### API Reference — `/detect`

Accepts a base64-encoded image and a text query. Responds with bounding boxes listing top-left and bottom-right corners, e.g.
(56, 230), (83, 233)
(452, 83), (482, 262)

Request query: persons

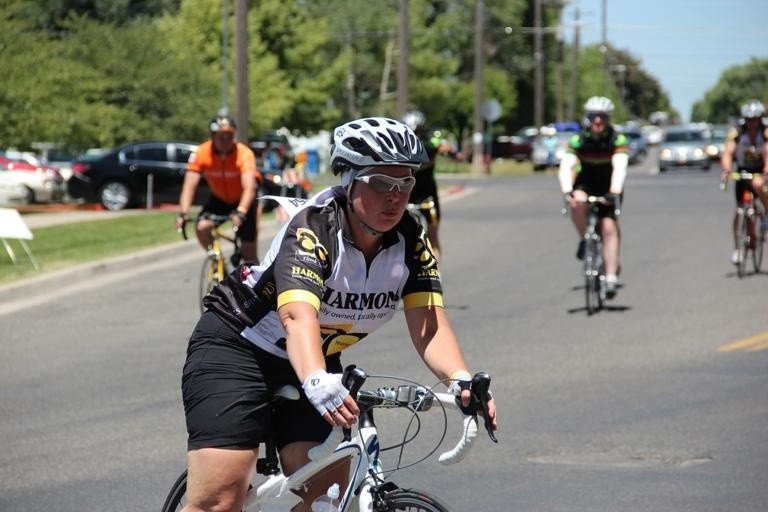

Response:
(399, 110), (444, 283)
(178, 117), (497, 511)
(174, 112), (260, 267)
(556, 94), (630, 300)
(718, 98), (768, 265)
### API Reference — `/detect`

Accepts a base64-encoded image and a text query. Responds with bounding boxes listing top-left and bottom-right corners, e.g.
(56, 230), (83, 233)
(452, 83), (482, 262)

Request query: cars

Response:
(465, 118), (729, 175)
(67, 140), (306, 212)
(0, 147), (75, 210)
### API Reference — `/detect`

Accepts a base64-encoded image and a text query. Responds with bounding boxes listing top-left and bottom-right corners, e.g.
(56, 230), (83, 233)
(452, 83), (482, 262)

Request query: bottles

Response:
(309, 482), (343, 512)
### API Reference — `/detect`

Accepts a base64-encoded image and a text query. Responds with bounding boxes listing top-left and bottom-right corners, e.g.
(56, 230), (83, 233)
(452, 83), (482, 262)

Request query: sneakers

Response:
(577, 241), (586, 259)
(732, 250), (744, 264)
(605, 282), (617, 300)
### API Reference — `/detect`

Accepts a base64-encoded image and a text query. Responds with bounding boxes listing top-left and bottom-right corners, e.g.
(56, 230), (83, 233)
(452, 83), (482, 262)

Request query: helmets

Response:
(585, 96), (615, 114)
(329, 111), (430, 177)
(739, 100), (764, 119)
(210, 117), (237, 132)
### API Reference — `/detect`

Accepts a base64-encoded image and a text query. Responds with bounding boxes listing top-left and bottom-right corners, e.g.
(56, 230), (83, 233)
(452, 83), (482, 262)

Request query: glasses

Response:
(353, 175), (416, 195)
(587, 113), (608, 121)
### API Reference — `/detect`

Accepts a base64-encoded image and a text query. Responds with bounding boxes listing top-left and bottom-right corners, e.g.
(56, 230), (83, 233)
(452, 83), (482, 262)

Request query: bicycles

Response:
(179, 213), (242, 317)
(718, 169), (768, 279)
(162, 365), (497, 512)
(560, 194), (622, 316)
